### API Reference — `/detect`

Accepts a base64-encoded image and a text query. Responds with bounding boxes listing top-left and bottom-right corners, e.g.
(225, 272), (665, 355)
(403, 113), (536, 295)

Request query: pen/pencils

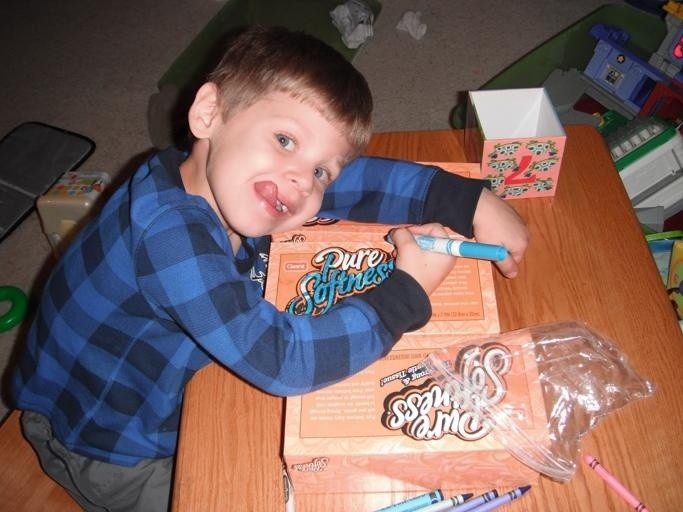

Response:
(384, 234), (508, 261)
(583, 454), (649, 512)
(374, 485), (531, 512)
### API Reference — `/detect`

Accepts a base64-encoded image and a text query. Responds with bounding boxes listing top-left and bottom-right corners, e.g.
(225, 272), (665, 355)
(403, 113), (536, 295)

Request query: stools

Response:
(0, 407), (87, 512)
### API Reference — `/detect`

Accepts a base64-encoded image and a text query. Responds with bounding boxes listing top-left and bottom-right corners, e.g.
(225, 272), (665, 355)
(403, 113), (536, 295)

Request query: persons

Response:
(1, 21), (531, 511)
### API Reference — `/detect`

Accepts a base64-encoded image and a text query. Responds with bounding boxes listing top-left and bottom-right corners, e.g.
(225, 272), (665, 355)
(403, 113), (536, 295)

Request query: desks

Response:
(170, 126), (683, 512)
